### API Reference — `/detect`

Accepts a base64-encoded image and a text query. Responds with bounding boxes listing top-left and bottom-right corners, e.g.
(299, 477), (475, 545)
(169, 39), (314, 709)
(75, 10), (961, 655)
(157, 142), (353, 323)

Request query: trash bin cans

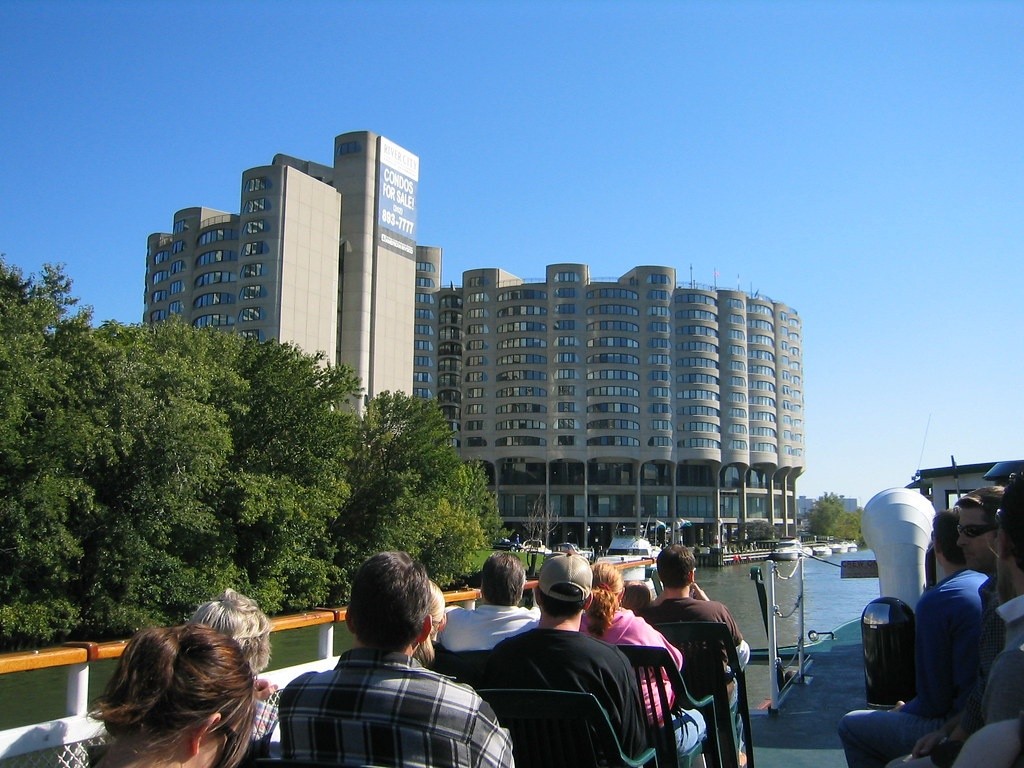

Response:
(860, 597), (916, 711)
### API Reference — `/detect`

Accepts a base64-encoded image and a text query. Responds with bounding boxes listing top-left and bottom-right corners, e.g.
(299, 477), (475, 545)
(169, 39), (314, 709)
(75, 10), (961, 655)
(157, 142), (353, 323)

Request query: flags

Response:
(656, 521), (667, 529)
(680, 519), (691, 527)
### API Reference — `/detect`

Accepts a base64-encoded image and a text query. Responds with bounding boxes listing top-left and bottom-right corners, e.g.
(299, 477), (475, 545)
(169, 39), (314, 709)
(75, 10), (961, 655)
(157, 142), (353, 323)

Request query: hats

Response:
(538, 549), (595, 603)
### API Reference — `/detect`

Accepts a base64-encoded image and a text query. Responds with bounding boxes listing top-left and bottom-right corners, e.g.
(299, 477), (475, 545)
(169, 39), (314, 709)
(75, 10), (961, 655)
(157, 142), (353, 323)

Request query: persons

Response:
(644, 544), (750, 768)
(622, 582), (651, 616)
(580, 562), (706, 755)
(278, 551), (515, 768)
(415, 579), (480, 689)
(91, 623), (255, 768)
(191, 589), (278, 756)
(836, 473), (1024, 768)
(480, 551), (648, 768)
(439, 551), (541, 651)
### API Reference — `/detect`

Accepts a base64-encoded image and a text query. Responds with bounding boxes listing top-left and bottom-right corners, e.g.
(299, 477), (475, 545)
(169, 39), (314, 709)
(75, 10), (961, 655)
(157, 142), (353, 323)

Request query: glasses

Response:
(930, 530), (943, 560)
(688, 567), (697, 575)
(956, 522), (999, 538)
(217, 722), (239, 767)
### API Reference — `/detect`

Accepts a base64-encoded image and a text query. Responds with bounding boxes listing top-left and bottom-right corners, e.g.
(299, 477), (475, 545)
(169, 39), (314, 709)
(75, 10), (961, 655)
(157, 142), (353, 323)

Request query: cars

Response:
(493, 537), (522, 553)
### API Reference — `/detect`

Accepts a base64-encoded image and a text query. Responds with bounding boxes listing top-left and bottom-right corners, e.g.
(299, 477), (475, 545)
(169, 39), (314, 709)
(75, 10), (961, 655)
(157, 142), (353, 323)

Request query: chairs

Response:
(475, 688), (659, 768)
(238, 758), (391, 768)
(613, 645), (723, 768)
(427, 650), (491, 690)
(652, 621), (754, 768)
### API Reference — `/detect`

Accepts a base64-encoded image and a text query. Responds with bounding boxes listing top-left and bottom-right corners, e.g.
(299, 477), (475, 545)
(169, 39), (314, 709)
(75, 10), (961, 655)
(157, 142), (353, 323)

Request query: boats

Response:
(829, 541), (858, 553)
(596, 536), (696, 589)
(523, 540), (552, 556)
(552, 542), (592, 559)
(771, 539), (812, 561)
(813, 543), (833, 556)
